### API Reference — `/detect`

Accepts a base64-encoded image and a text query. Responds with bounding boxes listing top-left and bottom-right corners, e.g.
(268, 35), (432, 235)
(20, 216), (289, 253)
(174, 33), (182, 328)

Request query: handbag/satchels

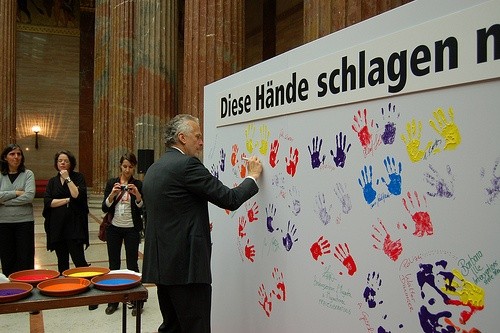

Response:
(98, 202), (117, 242)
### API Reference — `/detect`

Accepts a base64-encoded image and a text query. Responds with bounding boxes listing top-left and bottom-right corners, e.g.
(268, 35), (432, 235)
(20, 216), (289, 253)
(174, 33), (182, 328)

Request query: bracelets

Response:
(65, 198), (68, 204)
(65, 177), (71, 183)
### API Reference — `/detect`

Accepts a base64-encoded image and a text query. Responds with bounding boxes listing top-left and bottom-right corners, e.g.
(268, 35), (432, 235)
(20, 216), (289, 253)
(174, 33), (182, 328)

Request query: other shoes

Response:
(132, 305), (143, 316)
(89, 304), (99, 310)
(105, 305), (117, 315)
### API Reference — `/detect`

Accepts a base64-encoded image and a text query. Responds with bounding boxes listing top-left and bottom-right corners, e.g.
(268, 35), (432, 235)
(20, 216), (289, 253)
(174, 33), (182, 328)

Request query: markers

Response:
(241, 157), (248, 161)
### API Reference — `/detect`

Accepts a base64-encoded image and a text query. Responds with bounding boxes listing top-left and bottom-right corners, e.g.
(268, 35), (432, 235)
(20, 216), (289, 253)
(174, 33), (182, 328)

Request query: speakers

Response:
(137, 149), (154, 174)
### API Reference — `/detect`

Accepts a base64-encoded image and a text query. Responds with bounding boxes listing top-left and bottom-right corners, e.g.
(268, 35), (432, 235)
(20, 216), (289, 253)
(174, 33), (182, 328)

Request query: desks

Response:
(0, 276), (148, 333)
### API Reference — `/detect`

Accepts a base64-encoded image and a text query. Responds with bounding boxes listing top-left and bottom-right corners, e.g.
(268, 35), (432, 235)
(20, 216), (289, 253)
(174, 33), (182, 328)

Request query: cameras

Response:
(119, 185), (131, 192)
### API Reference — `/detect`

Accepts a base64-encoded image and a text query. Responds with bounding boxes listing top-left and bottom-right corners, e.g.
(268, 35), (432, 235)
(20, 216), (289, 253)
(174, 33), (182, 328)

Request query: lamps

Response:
(32, 126), (41, 149)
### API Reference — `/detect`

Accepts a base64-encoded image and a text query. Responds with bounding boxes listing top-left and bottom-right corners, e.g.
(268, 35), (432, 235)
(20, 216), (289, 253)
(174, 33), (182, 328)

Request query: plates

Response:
(9, 269), (60, 287)
(36, 278), (91, 297)
(62, 267), (110, 280)
(90, 274), (141, 290)
(0, 282), (33, 303)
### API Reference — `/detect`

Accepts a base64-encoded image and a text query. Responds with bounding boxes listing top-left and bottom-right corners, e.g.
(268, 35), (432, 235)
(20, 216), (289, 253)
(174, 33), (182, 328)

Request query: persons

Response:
(42, 150), (99, 310)
(102, 153), (143, 316)
(141, 113), (262, 333)
(0, 143), (41, 314)
